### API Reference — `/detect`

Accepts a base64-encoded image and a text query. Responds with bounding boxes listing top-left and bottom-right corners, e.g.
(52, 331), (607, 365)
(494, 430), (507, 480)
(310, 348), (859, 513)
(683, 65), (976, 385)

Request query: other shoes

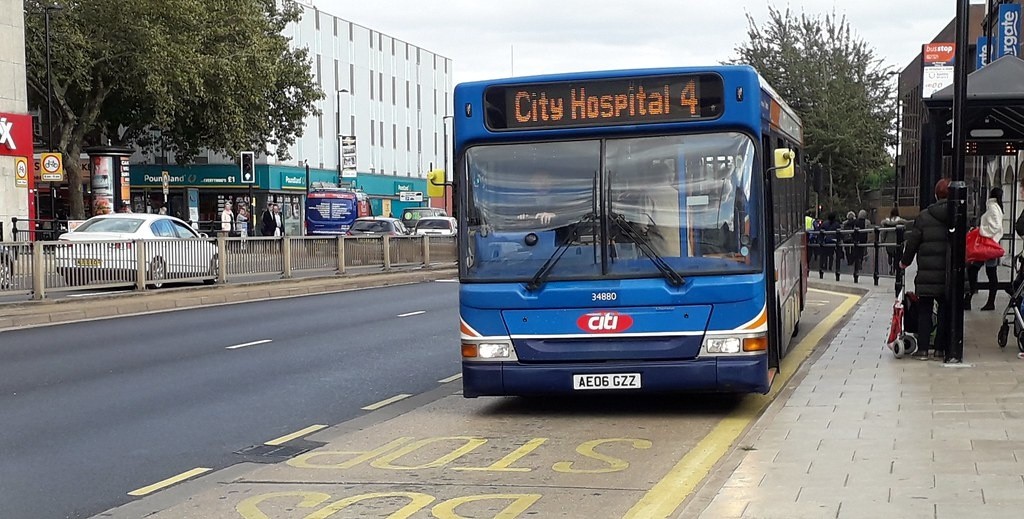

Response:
(934, 350), (944, 361)
(912, 350), (928, 361)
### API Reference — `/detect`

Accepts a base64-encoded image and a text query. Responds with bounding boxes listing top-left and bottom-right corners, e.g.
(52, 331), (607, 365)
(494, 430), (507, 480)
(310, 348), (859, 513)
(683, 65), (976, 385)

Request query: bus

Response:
(426, 62), (811, 400)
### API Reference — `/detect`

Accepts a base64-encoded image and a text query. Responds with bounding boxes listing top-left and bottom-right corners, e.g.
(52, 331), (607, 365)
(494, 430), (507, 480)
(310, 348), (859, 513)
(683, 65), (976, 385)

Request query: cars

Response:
(53, 211), (229, 290)
(0, 243), (15, 289)
(345, 215), (409, 236)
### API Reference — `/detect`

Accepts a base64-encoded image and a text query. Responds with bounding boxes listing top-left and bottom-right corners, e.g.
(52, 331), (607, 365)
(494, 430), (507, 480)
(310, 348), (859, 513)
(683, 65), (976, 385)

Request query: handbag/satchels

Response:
(965, 229), (1005, 262)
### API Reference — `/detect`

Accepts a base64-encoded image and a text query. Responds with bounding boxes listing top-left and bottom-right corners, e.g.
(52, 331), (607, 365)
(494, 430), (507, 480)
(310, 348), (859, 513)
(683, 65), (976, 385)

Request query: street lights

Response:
(337, 88), (350, 190)
(45, 4), (64, 250)
(442, 115), (453, 214)
(887, 70), (902, 222)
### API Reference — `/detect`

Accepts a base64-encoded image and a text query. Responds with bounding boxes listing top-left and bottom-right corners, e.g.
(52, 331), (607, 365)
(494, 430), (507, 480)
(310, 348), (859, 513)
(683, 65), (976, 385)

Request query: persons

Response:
(517, 169), (558, 224)
(805, 210), (819, 262)
(817, 211), (840, 271)
(237, 206), (250, 235)
(841, 209), (872, 265)
(882, 208), (902, 274)
(900, 178), (951, 360)
(220, 203), (235, 230)
(158, 207), (166, 215)
(964, 187), (1004, 311)
(263, 204), (285, 236)
(630, 162), (691, 256)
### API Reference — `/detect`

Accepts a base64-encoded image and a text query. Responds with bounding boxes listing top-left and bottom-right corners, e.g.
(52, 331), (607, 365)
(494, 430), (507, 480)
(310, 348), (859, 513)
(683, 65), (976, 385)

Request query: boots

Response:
(963, 262), (981, 310)
(980, 266), (998, 311)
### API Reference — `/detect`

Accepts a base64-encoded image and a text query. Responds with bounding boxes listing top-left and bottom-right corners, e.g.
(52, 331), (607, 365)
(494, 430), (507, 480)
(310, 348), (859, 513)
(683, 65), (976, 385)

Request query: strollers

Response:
(996, 248), (1024, 354)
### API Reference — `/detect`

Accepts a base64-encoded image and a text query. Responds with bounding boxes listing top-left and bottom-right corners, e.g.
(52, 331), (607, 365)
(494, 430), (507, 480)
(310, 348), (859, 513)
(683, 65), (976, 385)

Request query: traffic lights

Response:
(240, 151), (256, 183)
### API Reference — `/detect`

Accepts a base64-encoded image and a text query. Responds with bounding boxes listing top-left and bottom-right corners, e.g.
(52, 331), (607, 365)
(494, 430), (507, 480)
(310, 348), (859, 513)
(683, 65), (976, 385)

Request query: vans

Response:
(413, 216), (458, 236)
(400, 206), (447, 236)
